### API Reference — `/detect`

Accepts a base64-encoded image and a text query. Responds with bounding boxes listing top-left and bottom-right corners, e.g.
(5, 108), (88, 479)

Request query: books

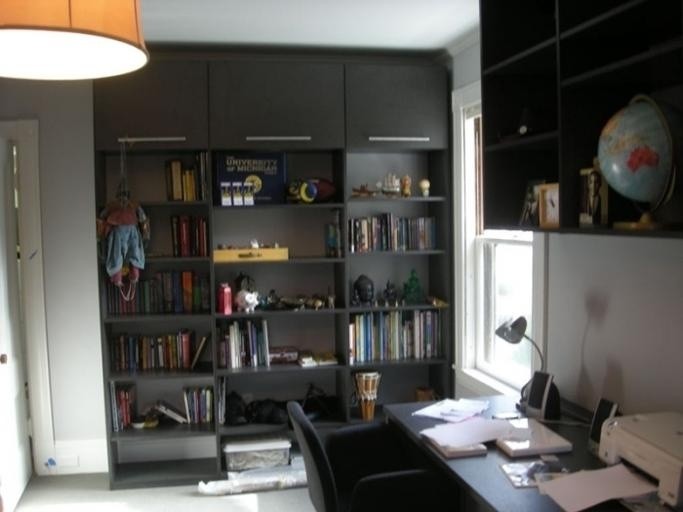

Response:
(216, 318), (271, 369)
(159, 155), (214, 429)
(424, 417), (487, 459)
(347, 308), (444, 368)
(348, 213), (438, 255)
(99, 269), (159, 434)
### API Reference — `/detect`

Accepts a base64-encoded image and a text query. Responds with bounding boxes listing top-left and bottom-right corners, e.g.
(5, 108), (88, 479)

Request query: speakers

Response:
(526, 372), (561, 421)
(587, 398), (622, 456)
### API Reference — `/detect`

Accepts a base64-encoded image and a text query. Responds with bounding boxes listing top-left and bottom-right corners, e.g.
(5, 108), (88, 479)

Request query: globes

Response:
(599, 94), (681, 231)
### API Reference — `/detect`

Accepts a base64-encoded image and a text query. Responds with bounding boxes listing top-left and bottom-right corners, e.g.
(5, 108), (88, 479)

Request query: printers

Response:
(599, 409), (682, 511)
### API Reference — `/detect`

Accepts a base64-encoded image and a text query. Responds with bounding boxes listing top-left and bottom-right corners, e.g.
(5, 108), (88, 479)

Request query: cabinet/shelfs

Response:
(478, 0), (683, 239)
(93, 51), (458, 490)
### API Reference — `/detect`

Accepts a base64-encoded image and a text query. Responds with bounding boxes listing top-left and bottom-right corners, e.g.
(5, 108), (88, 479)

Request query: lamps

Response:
(0, 1), (151, 84)
(494, 315), (548, 412)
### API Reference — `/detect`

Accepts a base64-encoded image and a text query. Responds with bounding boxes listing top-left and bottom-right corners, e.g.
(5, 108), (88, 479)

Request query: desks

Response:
(382, 397), (673, 512)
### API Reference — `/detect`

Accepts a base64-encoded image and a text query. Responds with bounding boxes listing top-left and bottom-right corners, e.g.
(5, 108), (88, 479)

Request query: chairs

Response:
(284, 400), (454, 512)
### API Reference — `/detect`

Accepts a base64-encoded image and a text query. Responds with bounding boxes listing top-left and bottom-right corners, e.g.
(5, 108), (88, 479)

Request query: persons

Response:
(582, 170), (602, 224)
(96, 180), (154, 302)
